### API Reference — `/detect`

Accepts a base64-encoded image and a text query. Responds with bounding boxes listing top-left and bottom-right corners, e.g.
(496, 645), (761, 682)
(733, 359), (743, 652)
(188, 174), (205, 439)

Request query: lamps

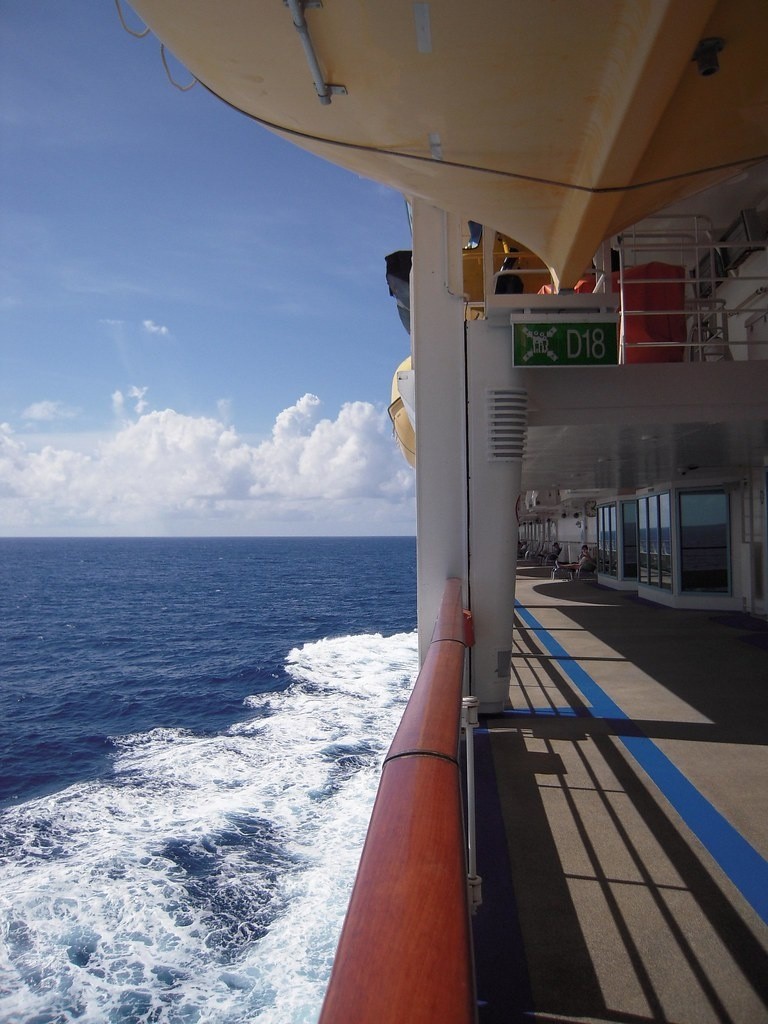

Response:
(692, 37), (723, 76)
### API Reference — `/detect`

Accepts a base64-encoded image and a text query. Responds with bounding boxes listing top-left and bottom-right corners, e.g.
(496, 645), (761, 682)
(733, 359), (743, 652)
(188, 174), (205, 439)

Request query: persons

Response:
(517, 540), (528, 556)
(537, 543), (561, 565)
(555, 545), (594, 570)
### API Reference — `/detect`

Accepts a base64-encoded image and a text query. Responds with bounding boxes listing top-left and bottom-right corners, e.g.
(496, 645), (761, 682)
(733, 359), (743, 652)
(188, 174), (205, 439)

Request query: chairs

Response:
(542, 553), (559, 566)
(551, 557), (597, 579)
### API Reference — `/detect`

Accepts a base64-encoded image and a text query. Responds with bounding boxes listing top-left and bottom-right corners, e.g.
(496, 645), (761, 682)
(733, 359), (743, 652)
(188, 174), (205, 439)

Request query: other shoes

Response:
(555, 560), (559, 569)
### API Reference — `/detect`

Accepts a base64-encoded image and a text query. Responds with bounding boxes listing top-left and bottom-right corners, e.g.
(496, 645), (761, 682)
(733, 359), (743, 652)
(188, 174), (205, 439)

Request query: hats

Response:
(552, 542), (558, 546)
